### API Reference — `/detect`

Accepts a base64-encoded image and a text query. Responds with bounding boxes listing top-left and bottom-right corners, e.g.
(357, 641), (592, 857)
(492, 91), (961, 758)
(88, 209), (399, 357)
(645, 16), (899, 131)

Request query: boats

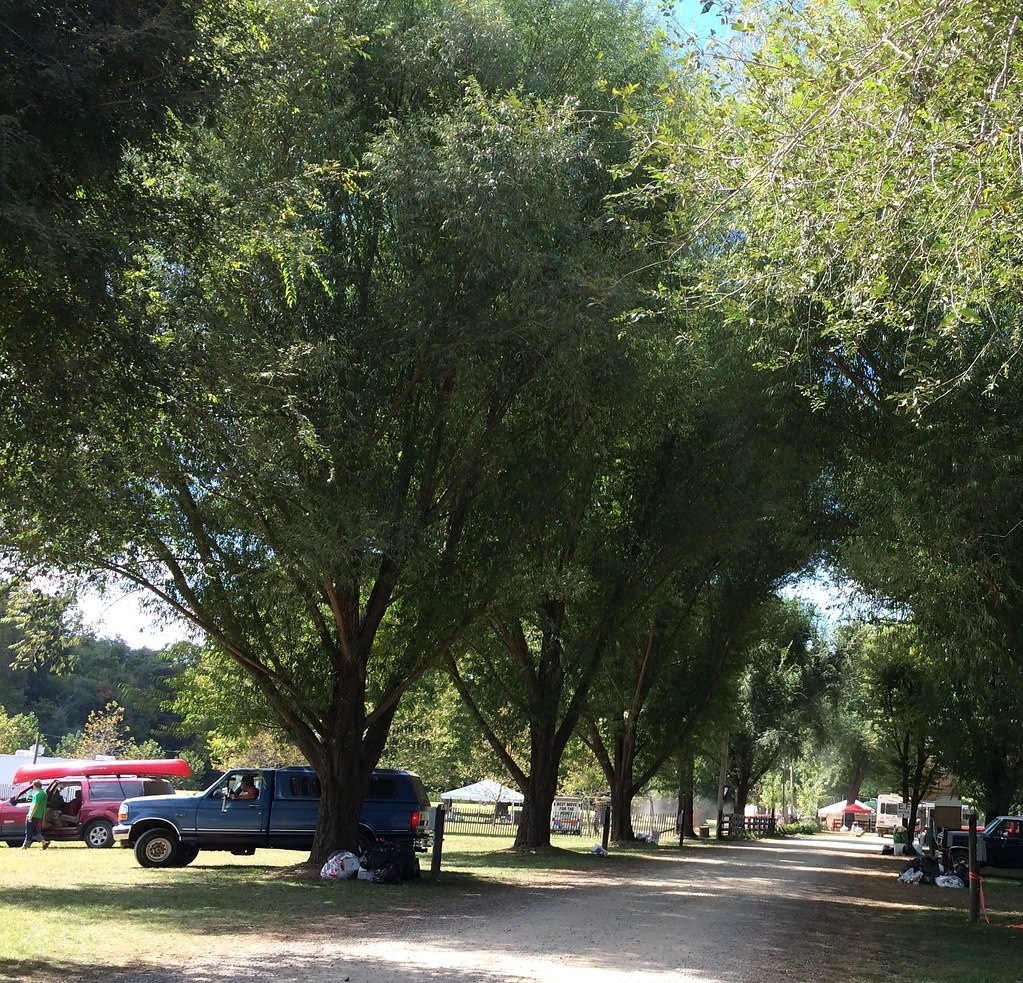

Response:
(12, 758), (191, 787)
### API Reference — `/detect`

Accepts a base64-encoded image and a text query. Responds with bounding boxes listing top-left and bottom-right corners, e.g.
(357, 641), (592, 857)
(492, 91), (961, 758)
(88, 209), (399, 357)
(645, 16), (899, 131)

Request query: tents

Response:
(818, 799), (875, 833)
(440, 779), (525, 825)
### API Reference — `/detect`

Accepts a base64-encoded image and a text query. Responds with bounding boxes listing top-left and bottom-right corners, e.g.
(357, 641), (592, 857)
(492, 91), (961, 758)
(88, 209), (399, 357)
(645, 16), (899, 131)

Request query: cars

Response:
(551, 811), (583, 835)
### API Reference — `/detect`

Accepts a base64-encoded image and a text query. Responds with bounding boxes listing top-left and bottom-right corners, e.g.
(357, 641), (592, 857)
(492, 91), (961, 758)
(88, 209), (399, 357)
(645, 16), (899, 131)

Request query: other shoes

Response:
(42, 841), (50, 850)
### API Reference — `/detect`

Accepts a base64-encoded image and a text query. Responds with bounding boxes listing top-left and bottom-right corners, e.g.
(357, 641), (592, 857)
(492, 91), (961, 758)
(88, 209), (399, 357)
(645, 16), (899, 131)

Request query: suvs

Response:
(0, 775), (176, 849)
(935, 815), (1023, 876)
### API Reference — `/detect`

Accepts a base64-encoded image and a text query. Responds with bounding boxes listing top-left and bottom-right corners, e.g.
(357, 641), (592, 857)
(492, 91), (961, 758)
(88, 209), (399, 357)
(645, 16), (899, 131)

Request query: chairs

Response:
(60, 809), (80, 827)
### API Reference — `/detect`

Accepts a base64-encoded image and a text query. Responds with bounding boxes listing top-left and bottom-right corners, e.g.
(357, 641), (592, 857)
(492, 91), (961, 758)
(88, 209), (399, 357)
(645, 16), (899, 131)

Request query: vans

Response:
(875, 792), (911, 837)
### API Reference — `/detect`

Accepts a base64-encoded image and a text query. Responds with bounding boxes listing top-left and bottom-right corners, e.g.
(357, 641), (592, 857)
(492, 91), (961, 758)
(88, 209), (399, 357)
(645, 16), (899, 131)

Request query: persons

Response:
(21, 778), (51, 850)
(1004, 821), (1019, 836)
(592, 810), (601, 838)
(232, 776), (257, 800)
(58, 789), (82, 816)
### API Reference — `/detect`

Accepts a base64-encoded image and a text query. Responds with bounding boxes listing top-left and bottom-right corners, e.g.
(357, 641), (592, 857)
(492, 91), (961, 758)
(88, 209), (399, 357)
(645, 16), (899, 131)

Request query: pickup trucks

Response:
(110, 763), (435, 871)
